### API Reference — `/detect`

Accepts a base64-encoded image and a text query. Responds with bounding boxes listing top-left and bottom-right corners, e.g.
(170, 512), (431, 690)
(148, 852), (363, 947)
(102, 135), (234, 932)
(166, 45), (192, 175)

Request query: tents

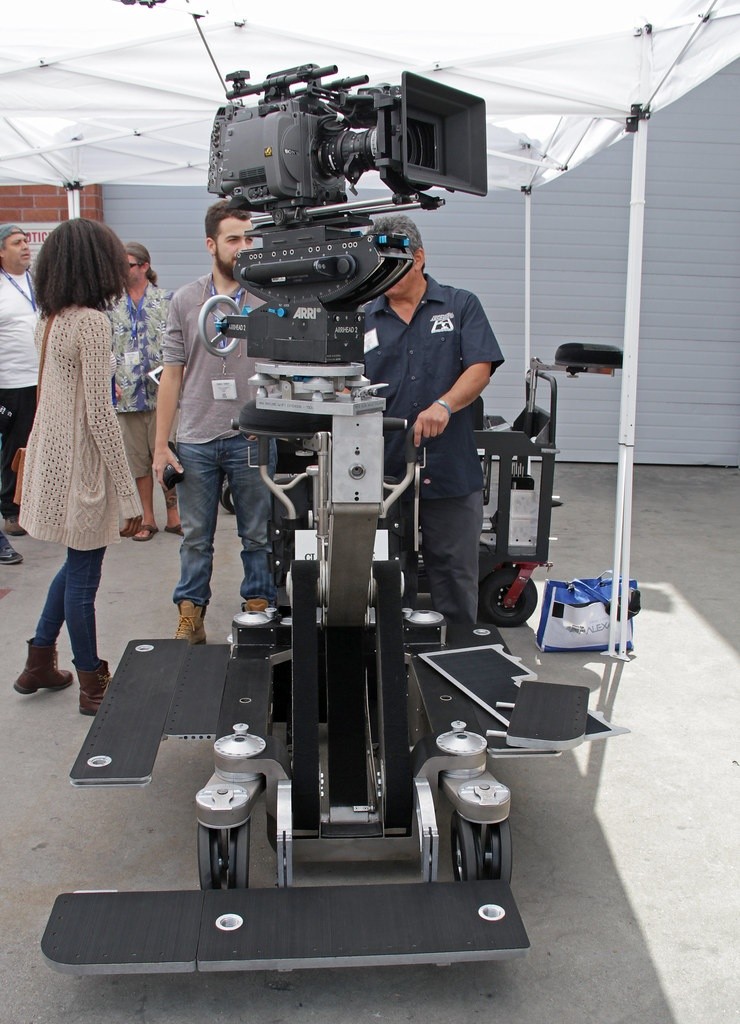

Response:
(0, 1), (740, 664)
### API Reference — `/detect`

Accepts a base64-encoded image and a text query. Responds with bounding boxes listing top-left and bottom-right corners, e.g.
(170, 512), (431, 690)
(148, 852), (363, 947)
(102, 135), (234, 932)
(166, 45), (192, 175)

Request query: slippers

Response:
(132, 525), (158, 541)
(164, 523), (184, 536)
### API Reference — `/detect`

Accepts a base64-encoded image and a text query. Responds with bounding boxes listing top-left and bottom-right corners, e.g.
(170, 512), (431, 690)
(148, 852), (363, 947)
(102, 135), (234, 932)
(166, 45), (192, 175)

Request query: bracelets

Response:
(434, 400), (451, 418)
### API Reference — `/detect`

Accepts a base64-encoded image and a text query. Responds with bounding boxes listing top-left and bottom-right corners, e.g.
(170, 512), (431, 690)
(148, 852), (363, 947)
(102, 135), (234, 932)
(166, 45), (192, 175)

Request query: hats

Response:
(0, 225), (25, 241)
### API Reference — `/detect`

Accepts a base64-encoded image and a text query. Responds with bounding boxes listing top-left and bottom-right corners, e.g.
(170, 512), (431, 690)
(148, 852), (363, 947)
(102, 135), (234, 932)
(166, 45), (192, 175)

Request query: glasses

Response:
(128, 262), (145, 267)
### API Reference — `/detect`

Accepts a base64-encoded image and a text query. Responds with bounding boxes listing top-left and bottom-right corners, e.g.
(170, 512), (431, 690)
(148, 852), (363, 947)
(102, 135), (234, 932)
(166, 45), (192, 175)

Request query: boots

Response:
(72, 659), (113, 716)
(13, 637), (73, 694)
(175, 601), (206, 645)
(247, 600), (268, 612)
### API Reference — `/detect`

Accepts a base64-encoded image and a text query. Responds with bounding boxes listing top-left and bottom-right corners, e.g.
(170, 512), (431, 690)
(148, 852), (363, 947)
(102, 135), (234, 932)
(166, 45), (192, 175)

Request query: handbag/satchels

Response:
(534, 570), (642, 652)
(11, 448), (27, 505)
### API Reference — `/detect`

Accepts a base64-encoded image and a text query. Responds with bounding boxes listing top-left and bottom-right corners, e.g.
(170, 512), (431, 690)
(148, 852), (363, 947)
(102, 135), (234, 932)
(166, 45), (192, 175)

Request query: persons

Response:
(13, 217), (145, 715)
(0, 225), (185, 565)
(360, 214), (505, 625)
(152, 200), (279, 646)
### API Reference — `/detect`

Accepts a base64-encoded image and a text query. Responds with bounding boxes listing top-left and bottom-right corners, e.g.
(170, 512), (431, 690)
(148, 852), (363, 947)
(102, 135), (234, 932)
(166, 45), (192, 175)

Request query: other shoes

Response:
(0, 544), (24, 564)
(5, 515), (27, 536)
